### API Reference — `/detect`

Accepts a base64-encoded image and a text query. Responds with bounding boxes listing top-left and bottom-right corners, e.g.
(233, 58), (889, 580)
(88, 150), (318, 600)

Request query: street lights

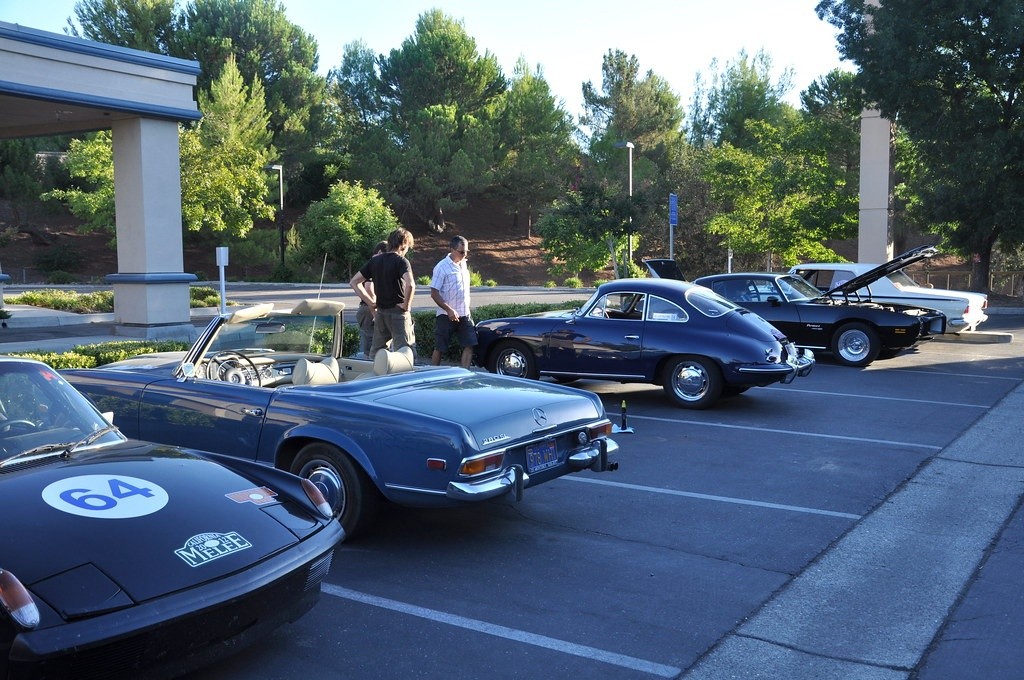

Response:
(265, 164), (284, 261)
(615, 142), (634, 263)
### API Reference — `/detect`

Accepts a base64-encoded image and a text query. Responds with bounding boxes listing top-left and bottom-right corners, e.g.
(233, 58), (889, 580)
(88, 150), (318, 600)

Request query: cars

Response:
(471, 278), (815, 409)
(0, 358), (346, 680)
(645, 245), (988, 367)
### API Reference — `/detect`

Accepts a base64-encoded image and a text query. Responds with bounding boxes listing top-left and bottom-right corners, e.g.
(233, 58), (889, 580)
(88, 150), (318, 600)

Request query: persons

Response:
(355, 240), (390, 360)
(428, 235), (479, 371)
(350, 228), (418, 366)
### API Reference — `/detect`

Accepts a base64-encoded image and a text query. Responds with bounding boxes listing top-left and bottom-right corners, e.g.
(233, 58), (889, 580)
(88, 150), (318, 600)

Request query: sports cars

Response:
(56, 299), (619, 536)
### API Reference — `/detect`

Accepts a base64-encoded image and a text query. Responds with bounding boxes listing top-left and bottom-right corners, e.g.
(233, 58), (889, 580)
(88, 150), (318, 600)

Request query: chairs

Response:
(354, 346), (414, 379)
(275, 356), (339, 390)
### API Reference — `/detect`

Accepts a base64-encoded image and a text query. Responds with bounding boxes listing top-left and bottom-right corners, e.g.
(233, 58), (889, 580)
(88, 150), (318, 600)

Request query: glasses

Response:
(452, 248), (471, 255)
